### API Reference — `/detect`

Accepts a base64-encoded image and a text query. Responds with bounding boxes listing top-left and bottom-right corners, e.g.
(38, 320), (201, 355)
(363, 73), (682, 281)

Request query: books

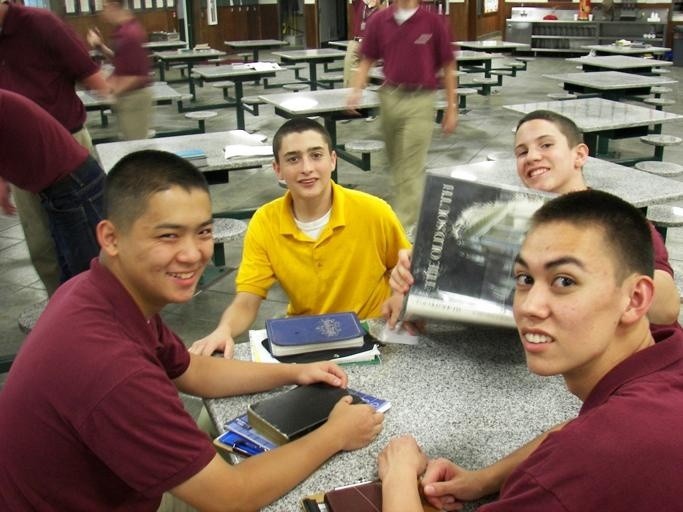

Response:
(224, 146), (274, 160)
(232, 61), (280, 73)
(177, 150), (209, 168)
(214, 312), (419, 511)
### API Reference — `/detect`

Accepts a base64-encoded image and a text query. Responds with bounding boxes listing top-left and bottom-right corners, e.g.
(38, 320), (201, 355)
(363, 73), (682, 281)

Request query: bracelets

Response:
(448, 103), (459, 108)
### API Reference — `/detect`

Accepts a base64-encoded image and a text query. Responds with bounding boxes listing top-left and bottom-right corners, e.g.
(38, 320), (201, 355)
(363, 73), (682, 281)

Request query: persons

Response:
(344, 1), (459, 231)
(88, 0), (155, 143)
(390, 110), (681, 330)
(0, 149), (384, 512)
(376, 191), (683, 512)
(0, 88), (104, 274)
(186, 116), (413, 360)
(343, 0), (389, 124)
(0, 1), (106, 298)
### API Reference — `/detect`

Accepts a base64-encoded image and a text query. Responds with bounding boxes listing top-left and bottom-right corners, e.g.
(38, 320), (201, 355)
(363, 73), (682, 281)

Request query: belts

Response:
(38, 154), (94, 200)
(385, 81), (437, 91)
(68, 123), (83, 133)
